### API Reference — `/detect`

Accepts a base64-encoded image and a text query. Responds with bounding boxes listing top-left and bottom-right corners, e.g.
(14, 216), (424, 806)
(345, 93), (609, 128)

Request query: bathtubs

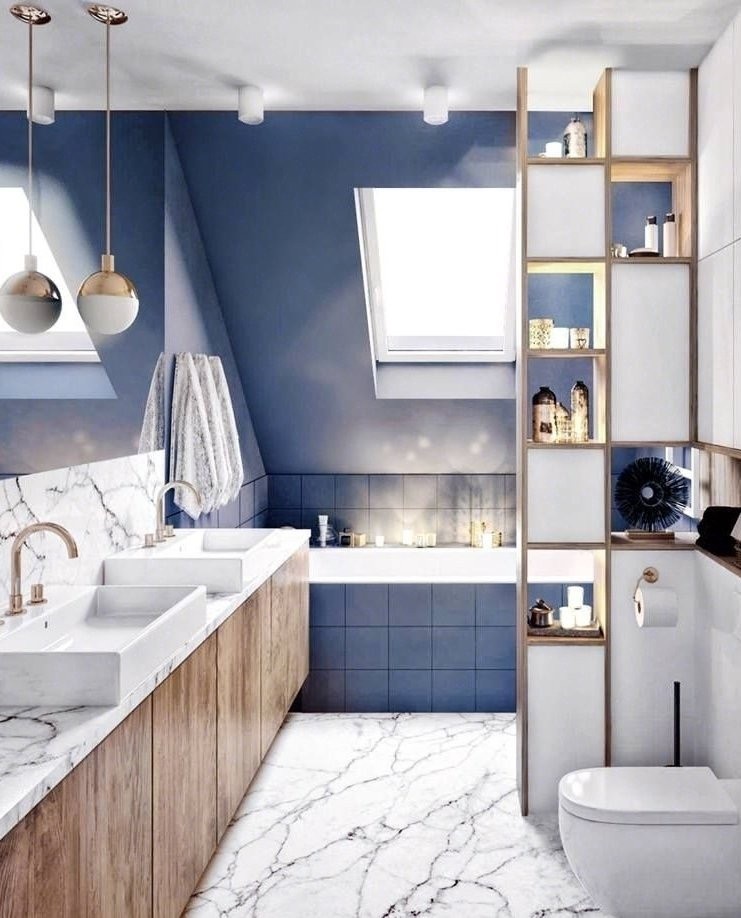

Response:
(290, 541), (598, 715)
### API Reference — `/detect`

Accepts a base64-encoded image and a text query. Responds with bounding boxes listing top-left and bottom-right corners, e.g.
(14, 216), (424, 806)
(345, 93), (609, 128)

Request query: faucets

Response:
(153, 480), (204, 543)
(5, 521), (81, 617)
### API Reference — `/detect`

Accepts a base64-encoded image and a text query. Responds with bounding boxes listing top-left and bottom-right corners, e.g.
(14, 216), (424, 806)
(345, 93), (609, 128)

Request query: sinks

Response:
(0, 581), (209, 709)
(103, 528), (283, 595)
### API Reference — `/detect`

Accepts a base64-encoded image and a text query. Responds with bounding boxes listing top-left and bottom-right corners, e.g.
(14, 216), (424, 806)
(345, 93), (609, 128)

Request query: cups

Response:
(614, 245), (627, 259)
(551, 327), (569, 349)
(416, 535), (425, 548)
(529, 319), (553, 350)
(545, 141), (562, 158)
(375, 536), (384, 546)
(426, 532), (436, 546)
(402, 529), (412, 545)
(559, 586), (591, 630)
(529, 599), (554, 628)
(570, 328), (590, 349)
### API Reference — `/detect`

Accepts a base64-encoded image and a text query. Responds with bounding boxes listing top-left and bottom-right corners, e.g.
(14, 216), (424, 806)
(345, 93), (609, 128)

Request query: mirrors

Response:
(0, 109), (166, 483)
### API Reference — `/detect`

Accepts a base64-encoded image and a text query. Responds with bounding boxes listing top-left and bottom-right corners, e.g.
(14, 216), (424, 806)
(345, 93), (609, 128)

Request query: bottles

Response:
(532, 384), (557, 444)
(555, 401), (571, 444)
(338, 527), (354, 548)
(562, 116), (588, 159)
(311, 524), (338, 547)
(571, 380), (589, 443)
(663, 213), (680, 258)
(644, 215), (659, 251)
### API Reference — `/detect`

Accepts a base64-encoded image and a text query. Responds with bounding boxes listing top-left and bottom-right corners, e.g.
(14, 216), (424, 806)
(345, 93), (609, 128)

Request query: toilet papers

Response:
(633, 588), (679, 628)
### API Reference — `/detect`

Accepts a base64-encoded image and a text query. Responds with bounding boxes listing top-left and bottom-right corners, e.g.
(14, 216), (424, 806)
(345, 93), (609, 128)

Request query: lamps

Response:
(28, 85), (55, 126)
(237, 84), (266, 127)
(77, 3), (140, 334)
(424, 84), (450, 126)
(0, 5), (63, 338)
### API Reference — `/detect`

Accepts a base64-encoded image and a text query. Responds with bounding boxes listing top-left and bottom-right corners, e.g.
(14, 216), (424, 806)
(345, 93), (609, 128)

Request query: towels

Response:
(170, 351), (245, 519)
(138, 350), (164, 454)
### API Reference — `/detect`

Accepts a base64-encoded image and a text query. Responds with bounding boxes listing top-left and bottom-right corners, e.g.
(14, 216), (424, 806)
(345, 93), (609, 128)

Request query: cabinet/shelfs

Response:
(0, 540), (312, 918)
(516, 63), (695, 817)
(693, 7), (741, 459)
(696, 546), (741, 781)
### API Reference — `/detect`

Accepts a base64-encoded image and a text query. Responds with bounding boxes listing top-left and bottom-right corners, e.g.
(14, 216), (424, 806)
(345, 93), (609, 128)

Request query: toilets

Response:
(555, 765), (741, 917)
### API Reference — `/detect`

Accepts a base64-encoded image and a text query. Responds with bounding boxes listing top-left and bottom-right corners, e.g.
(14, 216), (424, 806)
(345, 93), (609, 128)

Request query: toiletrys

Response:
(644, 215), (659, 250)
(563, 111), (588, 158)
(338, 527), (355, 548)
(318, 515), (329, 547)
(663, 213), (678, 257)
(532, 385), (558, 442)
